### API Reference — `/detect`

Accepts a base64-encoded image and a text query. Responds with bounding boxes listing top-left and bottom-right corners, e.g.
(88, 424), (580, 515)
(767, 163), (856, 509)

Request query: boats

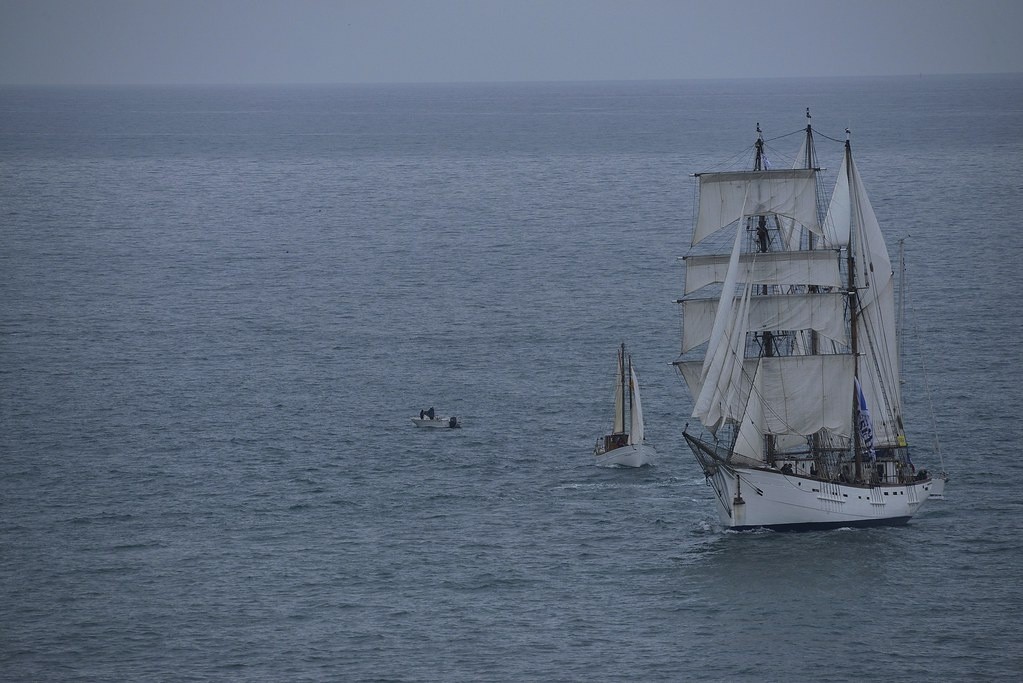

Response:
(411, 408), (461, 428)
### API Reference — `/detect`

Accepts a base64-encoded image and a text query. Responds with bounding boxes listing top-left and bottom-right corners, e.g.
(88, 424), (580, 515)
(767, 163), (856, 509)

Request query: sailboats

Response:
(893, 233), (952, 502)
(585, 342), (659, 467)
(669, 107), (934, 531)
